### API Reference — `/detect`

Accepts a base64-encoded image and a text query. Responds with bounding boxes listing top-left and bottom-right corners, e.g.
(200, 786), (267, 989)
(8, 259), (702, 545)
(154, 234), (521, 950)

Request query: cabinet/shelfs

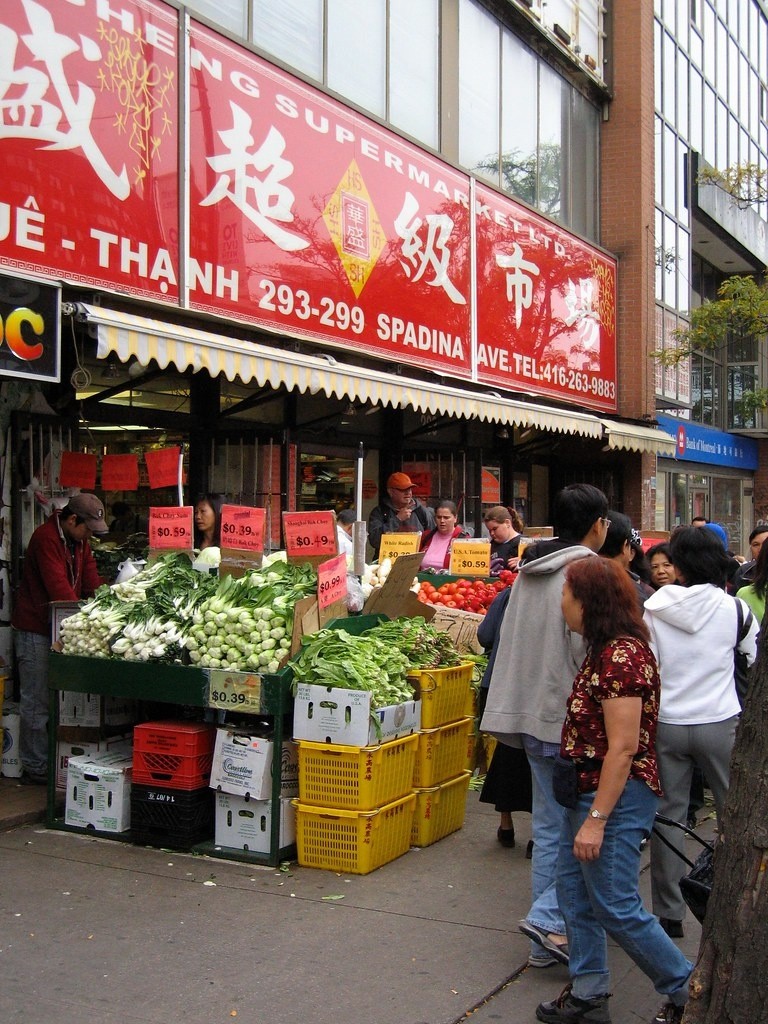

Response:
(45, 614), (393, 867)
(120, 441), (190, 519)
(296, 459), (358, 514)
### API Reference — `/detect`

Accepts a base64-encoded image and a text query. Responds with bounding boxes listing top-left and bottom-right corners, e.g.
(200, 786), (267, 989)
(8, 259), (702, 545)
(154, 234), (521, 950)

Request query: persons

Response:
(479, 484), (611, 968)
(735, 537), (768, 626)
(484, 506), (530, 571)
(418, 500), (471, 571)
(335, 509), (357, 556)
(110, 501), (147, 533)
(630, 528), (648, 579)
(477, 588), (532, 847)
(536, 556), (696, 1024)
(597, 510), (656, 614)
(194, 492), (231, 557)
(692, 517), (706, 527)
(731, 526), (768, 588)
(10, 492), (109, 785)
(645, 543), (680, 591)
(641, 526), (761, 938)
(368, 472), (436, 561)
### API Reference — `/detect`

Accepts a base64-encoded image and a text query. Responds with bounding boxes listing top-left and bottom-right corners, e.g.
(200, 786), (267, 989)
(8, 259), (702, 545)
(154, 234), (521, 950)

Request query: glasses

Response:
(735, 563), (757, 582)
(602, 518), (612, 528)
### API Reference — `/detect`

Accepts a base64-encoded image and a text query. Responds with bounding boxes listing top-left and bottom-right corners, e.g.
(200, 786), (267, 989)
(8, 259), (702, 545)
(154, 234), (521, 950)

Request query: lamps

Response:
(342, 403), (357, 416)
(128, 360), (148, 376)
(495, 428), (509, 439)
(518, 425), (531, 438)
(364, 406), (380, 415)
(101, 361), (119, 379)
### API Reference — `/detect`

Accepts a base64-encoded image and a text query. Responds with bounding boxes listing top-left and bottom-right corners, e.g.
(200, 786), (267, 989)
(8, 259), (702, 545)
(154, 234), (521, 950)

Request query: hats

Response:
(67, 494), (110, 532)
(338, 509), (357, 525)
(386, 472), (417, 490)
(703, 523), (728, 551)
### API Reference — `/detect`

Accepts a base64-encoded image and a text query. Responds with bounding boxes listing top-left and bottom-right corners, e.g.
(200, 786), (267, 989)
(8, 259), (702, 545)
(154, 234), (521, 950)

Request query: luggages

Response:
(650, 809), (721, 925)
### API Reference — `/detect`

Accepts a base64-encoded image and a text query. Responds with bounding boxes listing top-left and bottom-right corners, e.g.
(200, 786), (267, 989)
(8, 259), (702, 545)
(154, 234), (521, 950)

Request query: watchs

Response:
(589, 808), (608, 820)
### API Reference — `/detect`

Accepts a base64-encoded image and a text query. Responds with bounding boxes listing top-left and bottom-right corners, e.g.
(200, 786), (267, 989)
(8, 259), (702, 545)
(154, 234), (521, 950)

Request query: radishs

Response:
(363, 557), (421, 594)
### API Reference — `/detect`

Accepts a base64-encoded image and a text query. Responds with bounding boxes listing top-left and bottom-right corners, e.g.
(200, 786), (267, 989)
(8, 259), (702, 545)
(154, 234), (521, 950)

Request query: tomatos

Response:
(416, 578), (486, 608)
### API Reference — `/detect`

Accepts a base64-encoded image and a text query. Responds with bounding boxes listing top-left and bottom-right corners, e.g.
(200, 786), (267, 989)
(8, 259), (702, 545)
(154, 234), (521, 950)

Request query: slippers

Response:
(517, 918), (571, 967)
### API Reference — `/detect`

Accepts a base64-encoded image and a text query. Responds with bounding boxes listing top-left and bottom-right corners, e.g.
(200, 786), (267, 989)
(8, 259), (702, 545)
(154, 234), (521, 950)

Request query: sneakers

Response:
(655, 1003), (684, 1022)
(535, 984), (613, 1024)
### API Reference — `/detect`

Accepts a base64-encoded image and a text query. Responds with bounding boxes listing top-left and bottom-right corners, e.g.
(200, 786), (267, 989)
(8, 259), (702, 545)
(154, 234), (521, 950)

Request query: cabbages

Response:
(193, 547), (288, 568)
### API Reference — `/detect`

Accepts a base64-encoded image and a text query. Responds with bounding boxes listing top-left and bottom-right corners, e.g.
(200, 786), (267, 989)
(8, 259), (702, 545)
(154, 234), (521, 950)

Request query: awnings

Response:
(74, 301), (601, 440)
(601, 419), (677, 458)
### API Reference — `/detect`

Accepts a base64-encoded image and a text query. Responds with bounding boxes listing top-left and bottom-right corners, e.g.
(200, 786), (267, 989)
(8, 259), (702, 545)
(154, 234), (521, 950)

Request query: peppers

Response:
(453, 570), (518, 616)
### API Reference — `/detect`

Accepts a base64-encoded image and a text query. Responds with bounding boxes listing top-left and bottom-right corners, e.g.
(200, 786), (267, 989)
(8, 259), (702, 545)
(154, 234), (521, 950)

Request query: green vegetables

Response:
(60, 553), (319, 674)
(93, 532), (150, 579)
(287, 616), (489, 741)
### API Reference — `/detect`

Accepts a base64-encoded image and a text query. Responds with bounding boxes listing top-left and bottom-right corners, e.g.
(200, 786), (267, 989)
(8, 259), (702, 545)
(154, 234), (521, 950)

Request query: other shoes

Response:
(685, 815), (696, 835)
(657, 917), (686, 938)
(19, 771), (49, 786)
(526, 840), (535, 859)
(497, 825), (515, 848)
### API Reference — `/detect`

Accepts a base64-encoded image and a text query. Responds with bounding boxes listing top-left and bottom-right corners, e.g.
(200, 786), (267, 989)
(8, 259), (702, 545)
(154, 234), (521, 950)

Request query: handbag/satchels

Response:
(113, 557), (147, 585)
(551, 756), (579, 810)
(733, 597), (753, 697)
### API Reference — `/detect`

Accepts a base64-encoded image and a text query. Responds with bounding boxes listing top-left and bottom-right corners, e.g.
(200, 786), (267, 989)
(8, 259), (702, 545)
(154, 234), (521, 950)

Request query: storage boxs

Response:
(0, 598), (504, 875)
